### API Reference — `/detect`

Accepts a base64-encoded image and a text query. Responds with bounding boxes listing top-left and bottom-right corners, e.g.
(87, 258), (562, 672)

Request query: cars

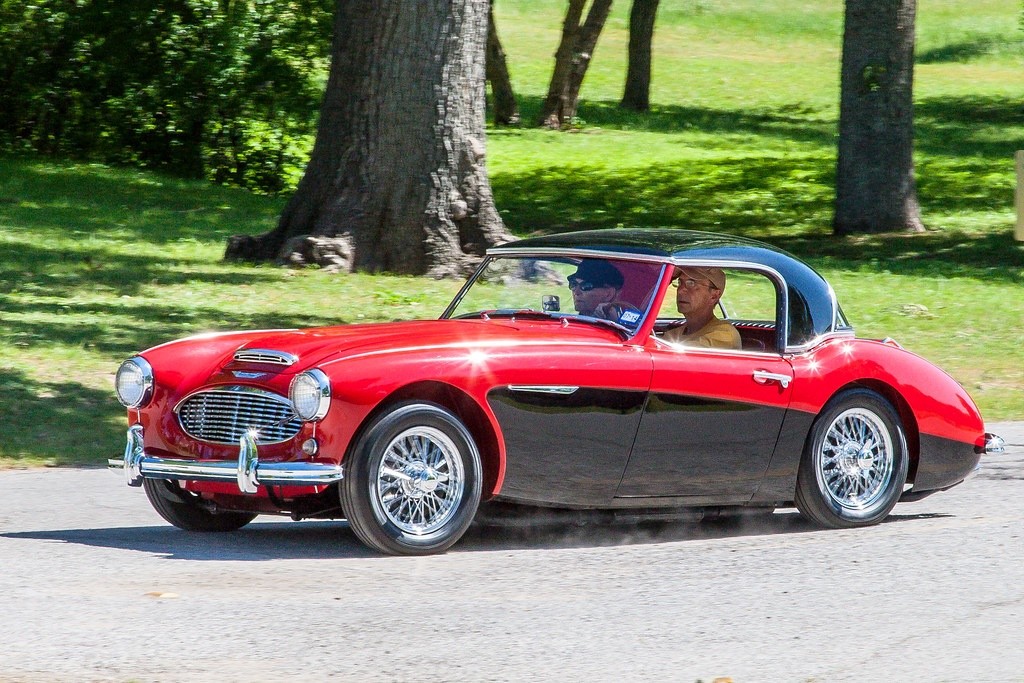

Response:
(109, 229), (1007, 558)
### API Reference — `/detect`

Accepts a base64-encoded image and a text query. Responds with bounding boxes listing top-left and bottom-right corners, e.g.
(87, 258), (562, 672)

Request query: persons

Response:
(566, 258), (625, 318)
(593, 266), (742, 351)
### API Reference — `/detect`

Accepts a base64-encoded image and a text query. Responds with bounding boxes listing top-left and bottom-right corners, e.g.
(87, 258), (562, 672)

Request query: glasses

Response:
(671, 279), (718, 289)
(568, 280), (609, 291)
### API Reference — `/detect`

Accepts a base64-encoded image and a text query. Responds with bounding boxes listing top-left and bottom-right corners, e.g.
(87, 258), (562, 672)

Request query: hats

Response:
(567, 258), (625, 290)
(673, 266), (726, 295)
